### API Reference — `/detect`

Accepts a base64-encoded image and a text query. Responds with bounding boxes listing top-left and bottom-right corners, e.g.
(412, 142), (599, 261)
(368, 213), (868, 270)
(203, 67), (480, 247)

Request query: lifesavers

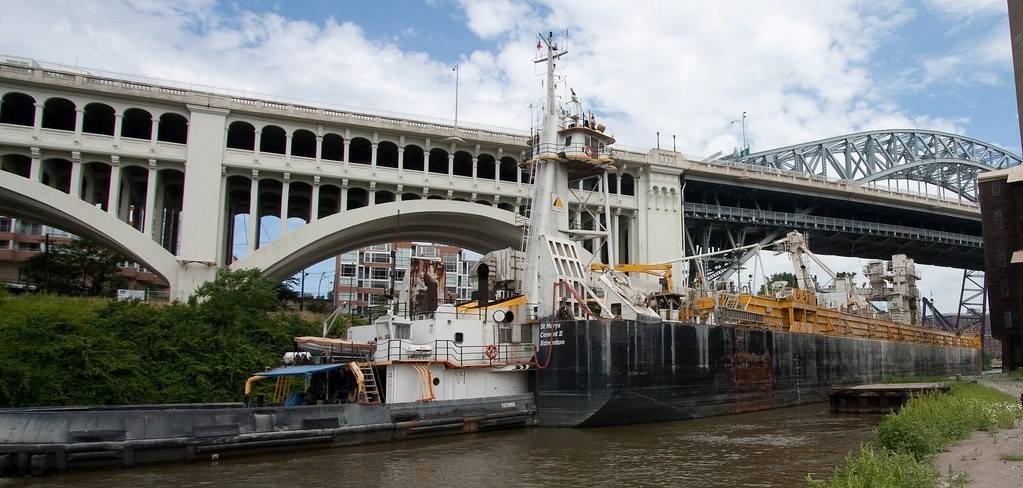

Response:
(486, 345), (497, 358)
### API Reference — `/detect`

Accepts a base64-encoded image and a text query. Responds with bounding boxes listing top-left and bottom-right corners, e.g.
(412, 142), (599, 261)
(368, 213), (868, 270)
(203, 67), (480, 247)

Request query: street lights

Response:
(453, 64), (458, 125)
(731, 112), (746, 163)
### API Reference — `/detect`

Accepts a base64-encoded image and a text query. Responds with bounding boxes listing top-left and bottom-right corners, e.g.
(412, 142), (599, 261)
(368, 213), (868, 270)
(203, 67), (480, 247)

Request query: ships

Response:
(244, 33), (981, 426)
